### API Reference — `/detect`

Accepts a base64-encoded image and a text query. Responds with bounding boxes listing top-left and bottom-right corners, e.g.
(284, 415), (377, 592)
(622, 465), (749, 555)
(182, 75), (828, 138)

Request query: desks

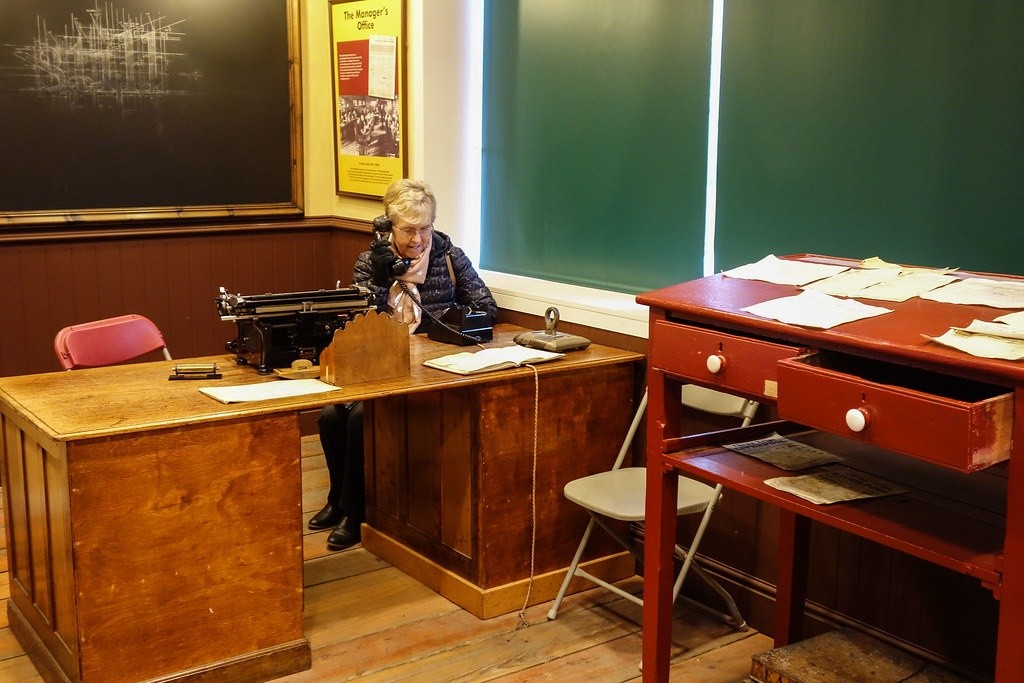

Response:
(0, 322), (646, 683)
(635, 254), (1024, 683)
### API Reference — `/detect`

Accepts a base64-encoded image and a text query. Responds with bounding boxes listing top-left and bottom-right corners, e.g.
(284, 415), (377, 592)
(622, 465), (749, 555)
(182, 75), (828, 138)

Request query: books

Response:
(420, 344), (567, 376)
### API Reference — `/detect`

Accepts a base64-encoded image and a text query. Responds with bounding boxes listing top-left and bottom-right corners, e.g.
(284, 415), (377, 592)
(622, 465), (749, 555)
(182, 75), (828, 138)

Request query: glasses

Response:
(393, 224), (434, 236)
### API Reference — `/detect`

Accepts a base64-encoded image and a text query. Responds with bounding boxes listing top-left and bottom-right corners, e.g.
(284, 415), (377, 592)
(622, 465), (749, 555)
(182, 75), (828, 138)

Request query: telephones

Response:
(372, 214), (411, 276)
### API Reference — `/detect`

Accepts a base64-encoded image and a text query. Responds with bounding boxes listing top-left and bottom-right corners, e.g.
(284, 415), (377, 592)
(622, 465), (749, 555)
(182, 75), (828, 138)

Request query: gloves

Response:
(370, 240), (407, 288)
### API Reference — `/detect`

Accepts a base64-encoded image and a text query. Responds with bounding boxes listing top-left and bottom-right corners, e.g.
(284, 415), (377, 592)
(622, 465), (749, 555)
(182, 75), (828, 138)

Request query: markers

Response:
(168, 374), (222, 380)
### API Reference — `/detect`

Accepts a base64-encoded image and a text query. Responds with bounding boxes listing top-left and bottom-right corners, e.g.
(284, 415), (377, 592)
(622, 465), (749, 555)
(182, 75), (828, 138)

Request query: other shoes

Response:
(327, 516), (365, 548)
(307, 504), (345, 533)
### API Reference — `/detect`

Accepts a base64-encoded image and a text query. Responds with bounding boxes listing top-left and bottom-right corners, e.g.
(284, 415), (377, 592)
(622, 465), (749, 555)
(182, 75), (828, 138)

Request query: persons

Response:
(352, 113), (369, 143)
(307, 179), (497, 549)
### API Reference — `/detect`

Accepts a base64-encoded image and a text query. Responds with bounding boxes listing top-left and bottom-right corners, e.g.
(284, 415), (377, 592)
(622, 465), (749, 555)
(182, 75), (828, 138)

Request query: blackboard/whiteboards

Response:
(0, 0), (307, 232)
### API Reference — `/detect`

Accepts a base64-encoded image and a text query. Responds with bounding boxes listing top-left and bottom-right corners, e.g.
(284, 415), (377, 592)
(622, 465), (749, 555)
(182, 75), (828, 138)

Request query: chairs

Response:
(545, 388), (760, 671)
(53, 313), (173, 372)
